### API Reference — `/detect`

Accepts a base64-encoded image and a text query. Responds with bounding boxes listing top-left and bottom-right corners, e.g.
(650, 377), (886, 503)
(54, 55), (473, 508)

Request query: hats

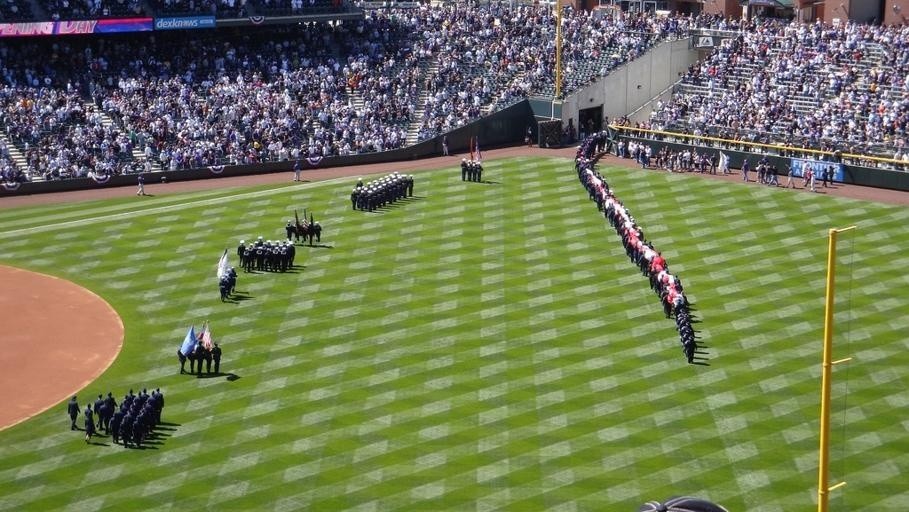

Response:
(641, 494), (732, 512)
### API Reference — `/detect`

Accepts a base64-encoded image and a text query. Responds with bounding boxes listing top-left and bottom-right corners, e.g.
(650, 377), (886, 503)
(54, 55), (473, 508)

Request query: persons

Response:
(177, 340), (222, 377)
(603, 135), (834, 191)
(68, 388), (165, 450)
(349, 172), (415, 211)
(416, 0), (724, 147)
(573, 129), (629, 211)
(217, 210), (323, 302)
(2, 0), (444, 196)
(442, 134), (484, 182)
(592, 211), (697, 364)
(695, 17), (909, 171)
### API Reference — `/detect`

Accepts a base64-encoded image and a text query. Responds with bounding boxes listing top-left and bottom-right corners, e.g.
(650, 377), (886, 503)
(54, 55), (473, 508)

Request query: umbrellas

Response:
(179, 322), (213, 356)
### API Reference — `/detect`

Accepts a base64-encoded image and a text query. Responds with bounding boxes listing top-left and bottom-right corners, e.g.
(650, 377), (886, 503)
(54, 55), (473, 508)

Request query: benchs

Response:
(652, 21), (909, 160)
